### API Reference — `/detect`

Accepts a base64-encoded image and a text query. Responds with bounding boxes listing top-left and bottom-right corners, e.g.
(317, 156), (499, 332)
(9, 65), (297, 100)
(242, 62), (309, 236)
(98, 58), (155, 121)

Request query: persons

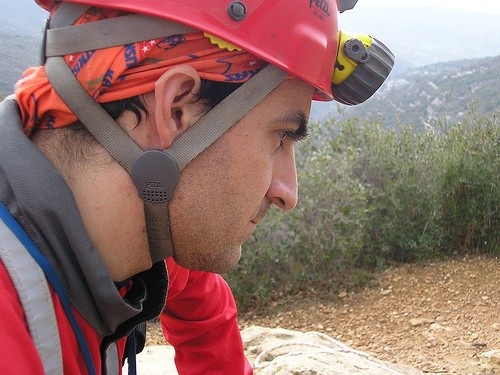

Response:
(0, 0), (318, 375)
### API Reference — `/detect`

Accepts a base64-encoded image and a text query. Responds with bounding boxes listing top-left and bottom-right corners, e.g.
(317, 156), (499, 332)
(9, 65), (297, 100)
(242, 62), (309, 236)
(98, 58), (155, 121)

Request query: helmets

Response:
(34, 0), (395, 106)
